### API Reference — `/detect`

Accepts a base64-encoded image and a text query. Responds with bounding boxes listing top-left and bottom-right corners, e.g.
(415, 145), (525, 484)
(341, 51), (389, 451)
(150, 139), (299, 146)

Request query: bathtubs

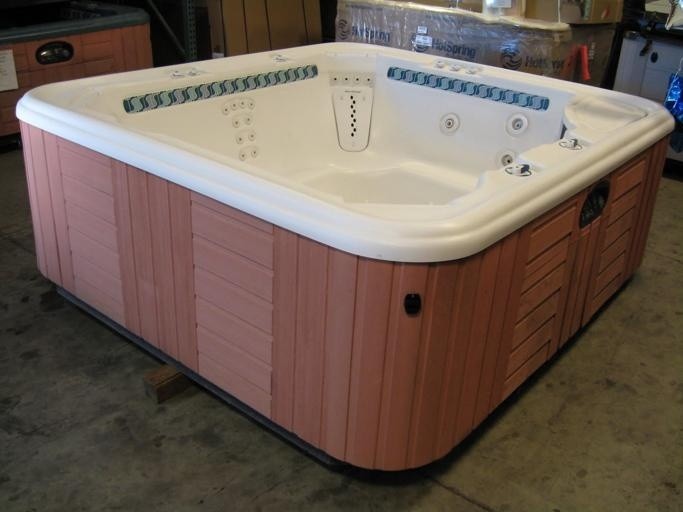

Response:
(15, 42), (676, 484)
